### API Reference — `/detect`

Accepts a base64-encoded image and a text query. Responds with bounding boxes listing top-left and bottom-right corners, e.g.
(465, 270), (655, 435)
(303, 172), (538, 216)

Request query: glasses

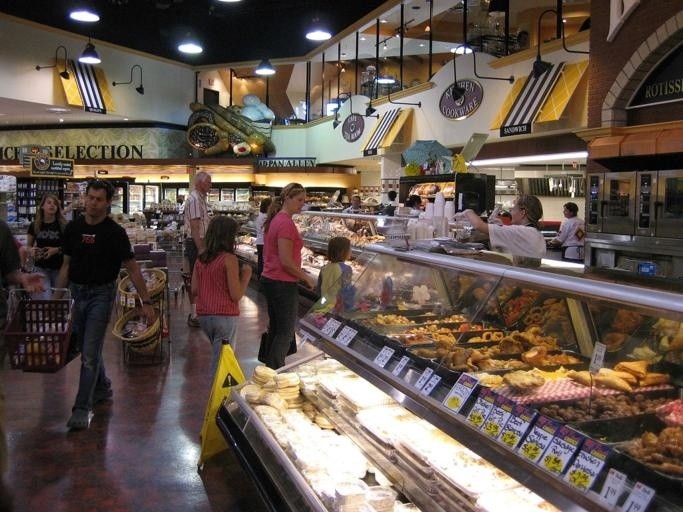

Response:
(365, 76), (422, 117)
(333, 92), (379, 129)
(68, 0), (101, 24)
(78, 32), (102, 66)
(254, 50), (277, 76)
(532, 9), (590, 78)
(176, 34), (204, 55)
(452, 44), (514, 102)
(112, 64), (145, 94)
(35, 45), (70, 80)
(303, 9), (333, 43)
(450, 30), (528, 55)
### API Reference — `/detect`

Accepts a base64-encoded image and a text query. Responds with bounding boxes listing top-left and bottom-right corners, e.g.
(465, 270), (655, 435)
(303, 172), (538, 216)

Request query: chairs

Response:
(67, 409), (88, 429)
(189, 314), (201, 326)
(93, 385), (112, 404)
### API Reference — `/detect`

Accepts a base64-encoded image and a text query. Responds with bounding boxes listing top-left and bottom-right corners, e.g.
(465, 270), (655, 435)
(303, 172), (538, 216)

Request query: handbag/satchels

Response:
(142, 299), (154, 305)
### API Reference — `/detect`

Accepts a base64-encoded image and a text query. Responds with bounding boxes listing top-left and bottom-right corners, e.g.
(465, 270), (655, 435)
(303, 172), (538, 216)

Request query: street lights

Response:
(258, 331), (296, 363)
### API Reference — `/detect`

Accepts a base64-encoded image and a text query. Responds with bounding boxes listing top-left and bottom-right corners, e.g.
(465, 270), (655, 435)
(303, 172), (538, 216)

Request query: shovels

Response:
(241, 366), (303, 410)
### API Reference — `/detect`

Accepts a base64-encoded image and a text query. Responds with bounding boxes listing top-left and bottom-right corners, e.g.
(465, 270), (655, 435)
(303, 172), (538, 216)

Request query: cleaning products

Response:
(155, 229), (186, 299)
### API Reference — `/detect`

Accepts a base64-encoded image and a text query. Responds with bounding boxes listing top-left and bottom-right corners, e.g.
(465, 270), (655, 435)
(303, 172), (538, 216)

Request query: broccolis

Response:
(110, 178), (252, 214)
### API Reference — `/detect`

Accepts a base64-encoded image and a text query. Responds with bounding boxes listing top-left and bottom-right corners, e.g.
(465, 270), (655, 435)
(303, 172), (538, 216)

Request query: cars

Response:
(407, 191), (455, 241)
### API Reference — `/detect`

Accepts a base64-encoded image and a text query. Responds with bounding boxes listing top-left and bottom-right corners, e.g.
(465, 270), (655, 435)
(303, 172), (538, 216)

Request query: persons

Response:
(183, 173), (212, 328)
(385, 190), (399, 210)
(548, 202), (585, 263)
(1, 218), (46, 489)
(342, 196), (362, 214)
(409, 195), (422, 210)
(190, 215), (253, 438)
(453, 195), (547, 257)
(45, 176), (155, 429)
(26, 193), (68, 298)
(316, 236), (357, 316)
(258, 181), (314, 366)
(256, 198), (272, 281)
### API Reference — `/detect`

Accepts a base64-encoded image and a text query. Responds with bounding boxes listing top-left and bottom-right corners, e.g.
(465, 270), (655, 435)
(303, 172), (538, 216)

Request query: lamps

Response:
(333, 92), (379, 129)
(78, 32), (102, 66)
(35, 45), (70, 80)
(365, 76), (422, 117)
(68, 0), (101, 24)
(303, 9), (333, 43)
(254, 50), (277, 76)
(176, 34), (204, 55)
(112, 64), (145, 94)
(450, 30), (528, 55)
(452, 44), (514, 102)
(532, 9), (590, 78)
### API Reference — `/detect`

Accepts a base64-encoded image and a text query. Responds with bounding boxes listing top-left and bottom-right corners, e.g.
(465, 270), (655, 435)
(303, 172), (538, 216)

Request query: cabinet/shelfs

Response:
(14, 176), (61, 220)
(217, 175), (683, 512)
(2, 170), (217, 291)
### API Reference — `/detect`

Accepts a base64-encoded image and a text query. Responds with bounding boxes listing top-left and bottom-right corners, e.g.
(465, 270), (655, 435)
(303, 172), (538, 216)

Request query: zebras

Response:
(582, 170), (682, 285)
